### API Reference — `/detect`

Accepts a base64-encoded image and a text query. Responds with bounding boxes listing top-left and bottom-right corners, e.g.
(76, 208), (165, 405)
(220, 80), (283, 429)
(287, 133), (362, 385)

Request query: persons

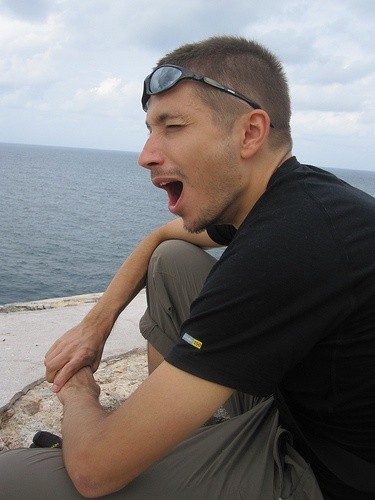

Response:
(0, 37), (374, 500)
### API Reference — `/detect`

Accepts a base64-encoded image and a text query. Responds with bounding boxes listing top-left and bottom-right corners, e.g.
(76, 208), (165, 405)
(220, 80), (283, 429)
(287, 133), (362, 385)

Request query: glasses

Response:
(141, 64), (274, 129)
